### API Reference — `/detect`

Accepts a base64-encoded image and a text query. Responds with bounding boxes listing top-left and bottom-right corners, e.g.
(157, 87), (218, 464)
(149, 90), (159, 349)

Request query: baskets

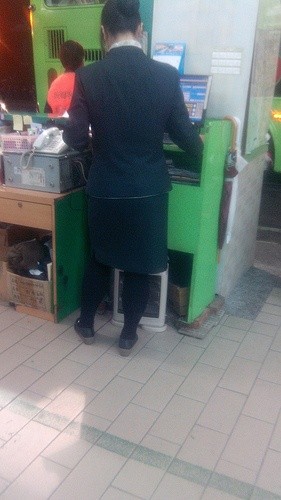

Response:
(1, 131), (37, 153)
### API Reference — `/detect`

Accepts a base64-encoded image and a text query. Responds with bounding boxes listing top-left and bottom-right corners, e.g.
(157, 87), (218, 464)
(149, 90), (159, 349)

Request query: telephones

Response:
(32, 125), (70, 155)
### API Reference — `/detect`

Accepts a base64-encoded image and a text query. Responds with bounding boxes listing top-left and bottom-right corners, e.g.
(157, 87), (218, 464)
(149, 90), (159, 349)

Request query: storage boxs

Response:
(6, 271), (54, 314)
(168, 280), (189, 315)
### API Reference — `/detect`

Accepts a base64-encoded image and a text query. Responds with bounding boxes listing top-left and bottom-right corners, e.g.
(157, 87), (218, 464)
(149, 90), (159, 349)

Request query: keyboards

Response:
(184, 102), (204, 119)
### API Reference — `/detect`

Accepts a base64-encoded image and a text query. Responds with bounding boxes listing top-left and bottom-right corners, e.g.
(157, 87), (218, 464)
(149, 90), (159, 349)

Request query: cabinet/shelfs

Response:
(0, 184), (88, 324)
(163, 118), (230, 323)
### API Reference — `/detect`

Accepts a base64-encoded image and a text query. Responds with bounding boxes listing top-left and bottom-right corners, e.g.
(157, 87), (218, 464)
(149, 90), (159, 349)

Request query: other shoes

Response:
(74, 317), (94, 345)
(119, 331), (138, 356)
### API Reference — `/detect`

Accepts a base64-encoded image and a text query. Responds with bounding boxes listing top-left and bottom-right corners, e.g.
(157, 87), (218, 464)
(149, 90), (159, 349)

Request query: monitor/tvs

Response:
(180, 74), (211, 109)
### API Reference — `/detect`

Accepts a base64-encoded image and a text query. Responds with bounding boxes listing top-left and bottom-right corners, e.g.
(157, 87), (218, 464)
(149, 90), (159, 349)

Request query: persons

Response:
(43, 39), (84, 113)
(61, 0), (203, 357)
